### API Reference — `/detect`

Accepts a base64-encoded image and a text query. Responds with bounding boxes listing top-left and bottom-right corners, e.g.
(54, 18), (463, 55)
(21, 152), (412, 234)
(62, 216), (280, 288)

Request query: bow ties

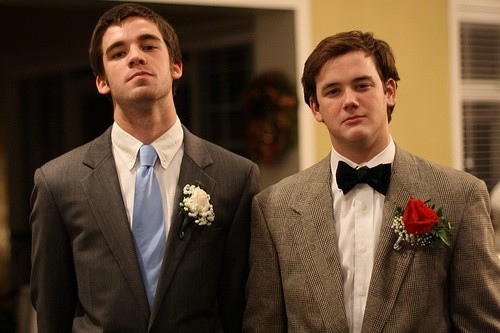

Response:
(336, 161), (392, 196)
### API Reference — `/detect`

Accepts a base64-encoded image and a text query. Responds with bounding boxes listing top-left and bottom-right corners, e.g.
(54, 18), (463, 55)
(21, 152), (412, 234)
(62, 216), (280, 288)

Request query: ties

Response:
(131, 144), (167, 315)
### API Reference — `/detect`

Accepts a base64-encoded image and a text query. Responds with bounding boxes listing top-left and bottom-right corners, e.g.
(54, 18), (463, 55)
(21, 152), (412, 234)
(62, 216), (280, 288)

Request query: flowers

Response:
(238, 70), (298, 161)
(391, 196), (454, 251)
(179, 180), (215, 239)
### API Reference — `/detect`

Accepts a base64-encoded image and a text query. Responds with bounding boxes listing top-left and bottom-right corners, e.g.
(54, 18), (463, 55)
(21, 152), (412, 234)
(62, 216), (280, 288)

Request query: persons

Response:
(28, 4), (262, 333)
(242, 31), (500, 332)
